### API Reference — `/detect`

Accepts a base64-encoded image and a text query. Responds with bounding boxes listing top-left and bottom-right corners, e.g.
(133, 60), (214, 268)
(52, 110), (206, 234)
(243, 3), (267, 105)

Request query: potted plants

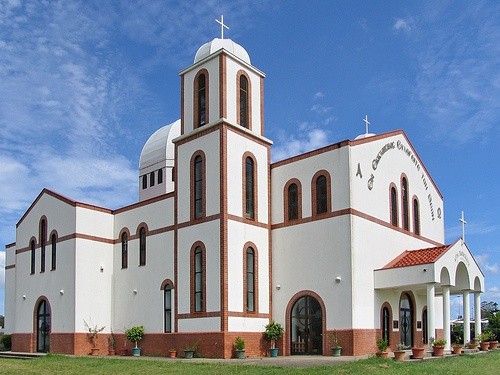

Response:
(392, 343), (406, 361)
(263, 320), (284, 356)
(183, 342), (199, 359)
(376, 338), (388, 359)
(168, 348), (177, 358)
(84, 320), (105, 356)
(126, 324), (143, 356)
(233, 337), (246, 359)
(453, 344), (462, 354)
(326, 332), (342, 357)
(432, 338), (447, 355)
(468, 331), (498, 351)
(108, 331), (116, 356)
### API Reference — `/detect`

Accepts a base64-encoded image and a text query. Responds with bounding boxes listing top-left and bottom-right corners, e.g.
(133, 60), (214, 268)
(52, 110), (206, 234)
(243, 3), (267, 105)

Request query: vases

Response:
(412, 348), (424, 359)
(120, 348), (128, 356)
